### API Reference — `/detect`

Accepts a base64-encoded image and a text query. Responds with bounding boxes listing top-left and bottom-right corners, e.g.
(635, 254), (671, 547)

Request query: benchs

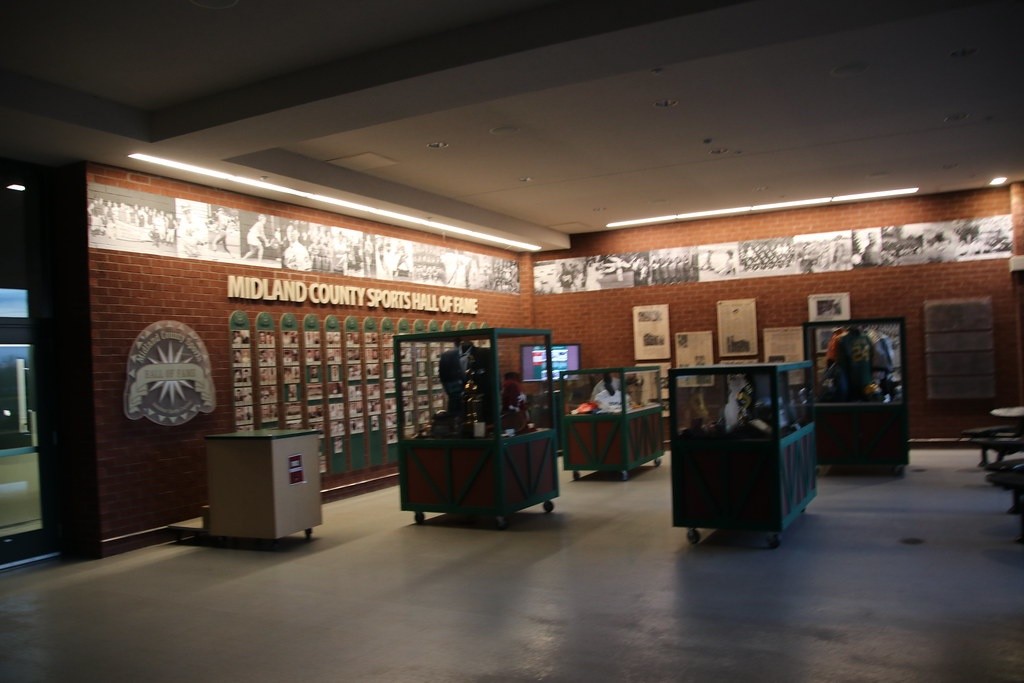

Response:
(962, 423), (1024, 466)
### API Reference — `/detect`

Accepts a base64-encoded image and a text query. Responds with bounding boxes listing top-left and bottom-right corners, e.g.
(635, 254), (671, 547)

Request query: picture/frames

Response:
(807, 292), (851, 322)
(716, 298), (758, 357)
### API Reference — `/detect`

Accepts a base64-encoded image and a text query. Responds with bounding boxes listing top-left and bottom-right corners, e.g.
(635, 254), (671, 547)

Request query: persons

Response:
(558, 262), (582, 292)
(232, 330), (490, 441)
(316, 227), (375, 278)
(584, 248), (738, 291)
(241, 214), (270, 264)
(178, 203), (237, 259)
(862, 232), (882, 265)
(825, 327), (895, 397)
(87, 196), (177, 248)
(437, 340), (495, 420)
(284, 222), (312, 271)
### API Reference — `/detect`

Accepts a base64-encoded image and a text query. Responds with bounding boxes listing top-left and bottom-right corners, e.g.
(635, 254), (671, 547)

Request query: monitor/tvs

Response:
(519, 343), (582, 383)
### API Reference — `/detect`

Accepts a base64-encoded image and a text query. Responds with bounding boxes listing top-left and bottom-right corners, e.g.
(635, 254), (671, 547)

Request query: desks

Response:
(988, 406), (1024, 470)
